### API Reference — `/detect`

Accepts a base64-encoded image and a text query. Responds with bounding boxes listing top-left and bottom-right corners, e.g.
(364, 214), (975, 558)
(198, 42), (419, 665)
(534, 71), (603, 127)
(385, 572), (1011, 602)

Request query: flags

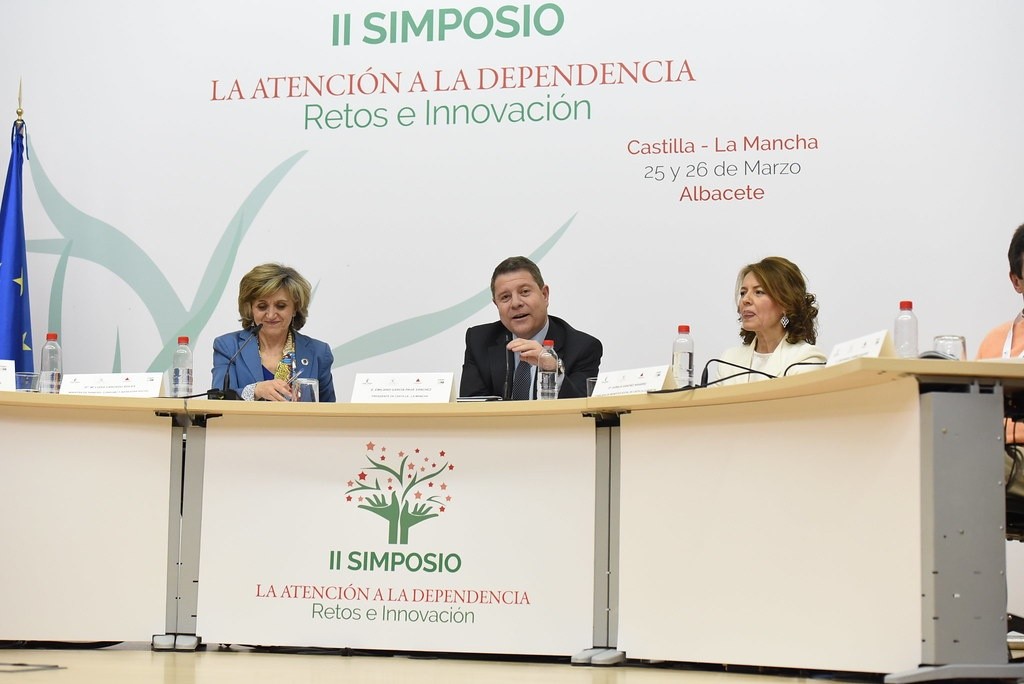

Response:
(0, 118), (36, 389)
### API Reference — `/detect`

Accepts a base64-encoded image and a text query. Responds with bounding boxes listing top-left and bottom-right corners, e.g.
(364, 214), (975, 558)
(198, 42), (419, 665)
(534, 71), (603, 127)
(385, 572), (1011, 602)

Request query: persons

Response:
(975, 224), (1024, 545)
(211, 263), (335, 403)
(715, 256), (828, 387)
(459, 256), (603, 401)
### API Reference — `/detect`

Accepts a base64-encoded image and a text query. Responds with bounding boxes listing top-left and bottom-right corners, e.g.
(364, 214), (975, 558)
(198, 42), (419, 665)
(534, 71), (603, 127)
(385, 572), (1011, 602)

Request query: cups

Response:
(933, 335), (967, 361)
(291, 377), (319, 403)
(15, 372), (40, 393)
(586, 377), (598, 397)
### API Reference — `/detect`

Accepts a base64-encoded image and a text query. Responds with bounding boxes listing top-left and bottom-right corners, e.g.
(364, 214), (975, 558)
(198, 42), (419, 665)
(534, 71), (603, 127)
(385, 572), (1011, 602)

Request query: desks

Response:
(0, 357), (1024, 680)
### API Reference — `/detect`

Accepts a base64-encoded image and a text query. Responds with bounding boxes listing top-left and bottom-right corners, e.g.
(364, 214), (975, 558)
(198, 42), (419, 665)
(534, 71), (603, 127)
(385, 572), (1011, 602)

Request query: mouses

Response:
(919, 351), (959, 361)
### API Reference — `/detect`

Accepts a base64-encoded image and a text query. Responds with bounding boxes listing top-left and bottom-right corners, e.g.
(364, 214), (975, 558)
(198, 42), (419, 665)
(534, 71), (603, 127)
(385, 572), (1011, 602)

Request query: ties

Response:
(513, 351), (531, 400)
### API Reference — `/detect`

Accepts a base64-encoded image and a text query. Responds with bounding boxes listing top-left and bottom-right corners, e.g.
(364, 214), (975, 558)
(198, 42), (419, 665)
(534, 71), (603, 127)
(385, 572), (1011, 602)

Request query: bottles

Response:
(893, 301), (919, 359)
(172, 336), (193, 398)
(40, 333), (63, 394)
(538, 340), (558, 401)
(672, 325), (696, 388)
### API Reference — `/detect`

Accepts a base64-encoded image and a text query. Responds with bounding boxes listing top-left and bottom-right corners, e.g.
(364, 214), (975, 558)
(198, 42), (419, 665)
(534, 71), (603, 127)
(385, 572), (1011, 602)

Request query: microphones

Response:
(701, 358), (778, 388)
(207, 323), (263, 400)
